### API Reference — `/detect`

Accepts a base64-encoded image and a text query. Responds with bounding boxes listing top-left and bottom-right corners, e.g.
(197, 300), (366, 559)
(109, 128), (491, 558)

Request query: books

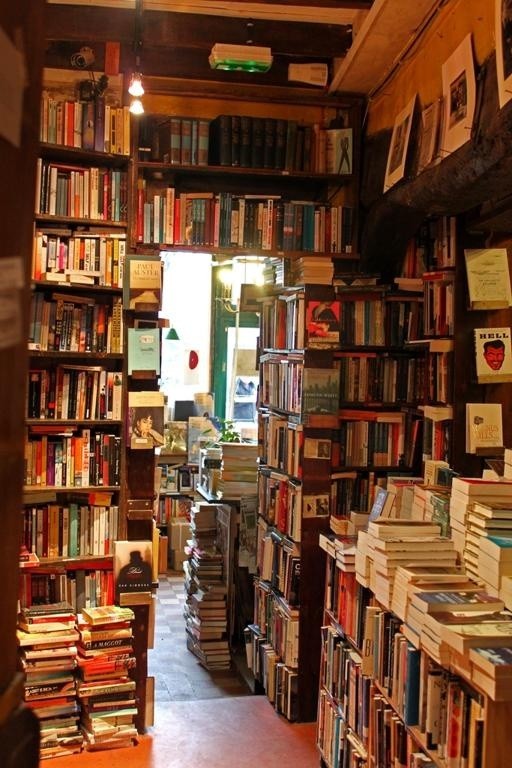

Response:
(181, 503), (233, 670)
(257, 340), (455, 428)
(196, 442), (258, 499)
(20, 490), (153, 596)
(31, 289), (162, 379)
(445, 476), (512, 612)
(258, 272), (457, 353)
(475, 327), (512, 384)
(255, 469), (300, 539)
(325, 558), (485, 767)
(138, 178), (356, 255)
(39, 93), (131, 156)
(465, 403), (503, 453)
(187, 416), (223, 464)
(354, 519), (512, 702)
(33, 228), (163, 313)
(320, 473), (453, 572)
(254, 580), (298, 668)
(292, 218), (459, 290)
(34, 156), (127, 221)
(319, 626), (432, 766)
(158, 464), (198, 493)
(16, 575), (138, 759)
(466, 248), (512, 308)
(245, 624), (298, 723)
(23, 426), (123, 489)
(255, 409), (454, 476)
(24, 364), (164, 450)
(157, 496), (194, 523)
(315, 693), (371, 766)
(138, 112), (354, 175)
(255, 521), (301, 605)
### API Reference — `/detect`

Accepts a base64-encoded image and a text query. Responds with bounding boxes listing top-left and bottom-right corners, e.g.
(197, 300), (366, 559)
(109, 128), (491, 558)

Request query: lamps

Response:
(129, 1), (146, 116)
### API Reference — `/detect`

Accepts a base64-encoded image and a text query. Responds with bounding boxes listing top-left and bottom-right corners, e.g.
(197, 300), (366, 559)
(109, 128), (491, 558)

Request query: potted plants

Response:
(200, 417), (252, 469)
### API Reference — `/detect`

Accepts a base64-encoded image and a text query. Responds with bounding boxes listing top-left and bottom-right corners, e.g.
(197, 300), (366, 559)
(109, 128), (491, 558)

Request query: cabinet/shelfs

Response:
(127, 11), (370, 260)
(317, 552), (512, 768)
(0, 0), (159, 768)
(246, 285), (435, 722)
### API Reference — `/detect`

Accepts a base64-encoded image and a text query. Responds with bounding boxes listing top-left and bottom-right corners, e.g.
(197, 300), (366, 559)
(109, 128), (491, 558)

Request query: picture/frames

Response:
(440, 34), (476, 161)
(495, 1), (512, 109)
(383, 93), (417, 193)
(416, 99), (439, 177)
